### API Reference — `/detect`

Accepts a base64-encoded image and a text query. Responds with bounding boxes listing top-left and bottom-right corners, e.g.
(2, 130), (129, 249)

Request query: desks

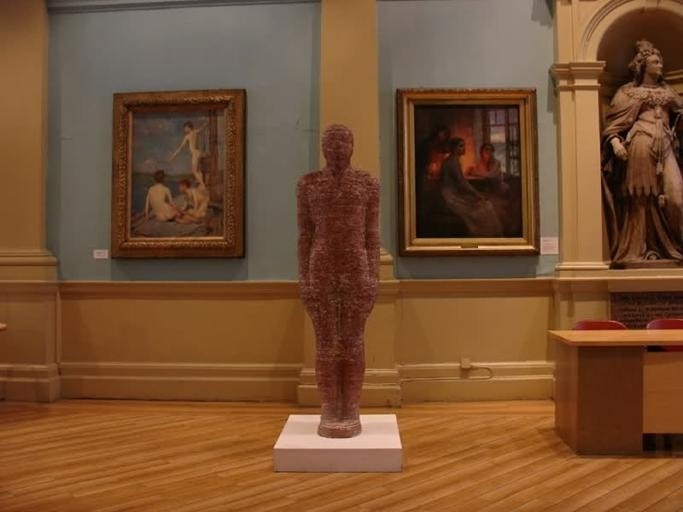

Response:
(546, 327), (683, 456)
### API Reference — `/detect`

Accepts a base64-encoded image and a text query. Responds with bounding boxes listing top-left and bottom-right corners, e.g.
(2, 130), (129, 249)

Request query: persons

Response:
(143, 120), (210, 223)
(295, 123), (381, 438)
(605, 38), (682, 267)
(422, 125), (511, 238)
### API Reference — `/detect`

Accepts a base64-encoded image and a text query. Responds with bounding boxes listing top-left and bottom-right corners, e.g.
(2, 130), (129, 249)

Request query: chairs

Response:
(570, 318), (683, 331)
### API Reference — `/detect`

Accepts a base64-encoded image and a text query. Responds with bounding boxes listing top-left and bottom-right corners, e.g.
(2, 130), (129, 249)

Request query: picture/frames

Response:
(111, 88), (248, 260)
(394, 87), (543, 258)
